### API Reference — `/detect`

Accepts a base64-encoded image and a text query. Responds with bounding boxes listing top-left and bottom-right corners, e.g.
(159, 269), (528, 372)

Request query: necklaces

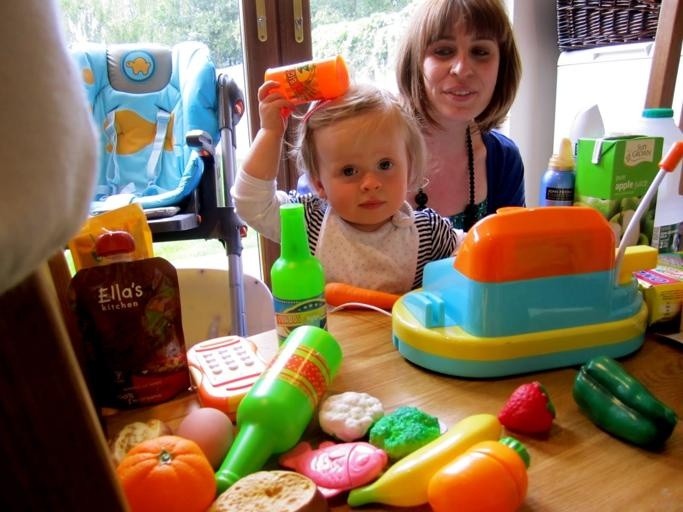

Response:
(413, 124), (478, 232)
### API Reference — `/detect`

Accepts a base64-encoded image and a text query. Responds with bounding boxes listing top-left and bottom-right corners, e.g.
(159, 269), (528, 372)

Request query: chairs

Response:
(68, 40), (249, 342)
(175, 269), (275, 353)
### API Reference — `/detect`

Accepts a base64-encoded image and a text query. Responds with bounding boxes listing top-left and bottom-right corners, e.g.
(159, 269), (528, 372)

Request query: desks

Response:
(101, 308), (683, 511)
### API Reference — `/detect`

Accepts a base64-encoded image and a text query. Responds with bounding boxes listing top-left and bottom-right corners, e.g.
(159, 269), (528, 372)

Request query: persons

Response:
(228, 80), (469, 308)
(391, 0), (526, 238)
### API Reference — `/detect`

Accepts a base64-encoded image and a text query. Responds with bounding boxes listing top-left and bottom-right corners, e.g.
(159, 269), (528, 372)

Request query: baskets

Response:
(555, 0), (661, 52)
